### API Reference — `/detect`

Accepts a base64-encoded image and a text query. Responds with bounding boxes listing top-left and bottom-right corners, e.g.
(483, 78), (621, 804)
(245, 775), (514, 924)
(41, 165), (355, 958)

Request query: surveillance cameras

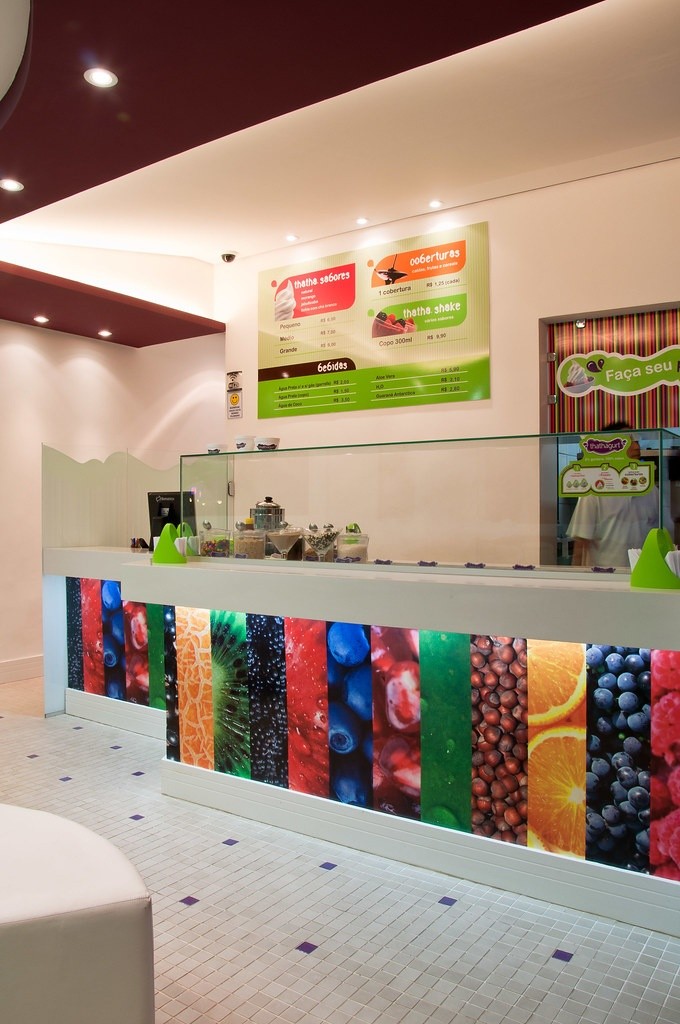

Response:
(220, 251), (236, 262)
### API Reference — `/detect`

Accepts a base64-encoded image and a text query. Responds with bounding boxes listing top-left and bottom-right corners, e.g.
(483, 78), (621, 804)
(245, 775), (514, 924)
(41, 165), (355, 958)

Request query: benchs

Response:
(0, 803), (156, 1024)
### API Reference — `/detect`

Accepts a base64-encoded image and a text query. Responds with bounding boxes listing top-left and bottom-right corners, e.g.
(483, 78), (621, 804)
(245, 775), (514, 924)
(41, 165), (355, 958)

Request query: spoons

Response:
(203, 520), (211, 530)
(279, 521), (288, 528)
(323, 523), (334, 528)
(235, 520), (246, 531)
(309, 523), (318, 532)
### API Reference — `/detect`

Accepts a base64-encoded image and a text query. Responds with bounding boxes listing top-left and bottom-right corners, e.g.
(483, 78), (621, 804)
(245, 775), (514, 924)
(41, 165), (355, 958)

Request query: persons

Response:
(565, 422), (660, 567)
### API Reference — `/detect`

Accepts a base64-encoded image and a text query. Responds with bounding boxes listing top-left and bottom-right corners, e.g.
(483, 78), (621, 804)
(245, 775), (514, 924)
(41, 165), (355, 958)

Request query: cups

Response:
(337, 533), (369, 561)
(234, 529), (265, 559)
(199, 528), (231, 558)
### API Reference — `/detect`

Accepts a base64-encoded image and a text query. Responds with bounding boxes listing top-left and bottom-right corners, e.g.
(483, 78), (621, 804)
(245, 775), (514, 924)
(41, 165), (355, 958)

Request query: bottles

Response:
(245, 518), (254, 531)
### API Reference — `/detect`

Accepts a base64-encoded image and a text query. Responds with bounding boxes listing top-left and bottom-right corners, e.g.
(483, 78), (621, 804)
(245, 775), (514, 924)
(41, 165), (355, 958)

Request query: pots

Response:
(249, 497), (285, 530)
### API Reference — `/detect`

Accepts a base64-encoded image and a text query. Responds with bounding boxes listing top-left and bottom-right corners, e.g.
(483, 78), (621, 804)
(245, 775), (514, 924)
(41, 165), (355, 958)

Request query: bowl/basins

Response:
(254, 438), (280, 451)
(232, 436), (255, 451)
(207, 443), (227, 454)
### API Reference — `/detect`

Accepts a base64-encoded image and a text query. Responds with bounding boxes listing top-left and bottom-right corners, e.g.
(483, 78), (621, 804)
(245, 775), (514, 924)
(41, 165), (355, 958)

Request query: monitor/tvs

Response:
(148, 491), (198, 551)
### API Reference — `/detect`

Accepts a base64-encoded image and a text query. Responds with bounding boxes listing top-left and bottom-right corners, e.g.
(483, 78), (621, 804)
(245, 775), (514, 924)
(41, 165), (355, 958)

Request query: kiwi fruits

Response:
(210, 610), (251, 780)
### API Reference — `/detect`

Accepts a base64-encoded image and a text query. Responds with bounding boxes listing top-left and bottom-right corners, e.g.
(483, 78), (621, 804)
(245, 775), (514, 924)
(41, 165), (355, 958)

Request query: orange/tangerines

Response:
(526, 640), (586, 860)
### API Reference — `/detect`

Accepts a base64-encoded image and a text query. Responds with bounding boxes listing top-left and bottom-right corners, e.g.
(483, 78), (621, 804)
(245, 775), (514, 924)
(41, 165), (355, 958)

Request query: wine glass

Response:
(266, 528), (304, 560)
(301, 528), (344, 562)
